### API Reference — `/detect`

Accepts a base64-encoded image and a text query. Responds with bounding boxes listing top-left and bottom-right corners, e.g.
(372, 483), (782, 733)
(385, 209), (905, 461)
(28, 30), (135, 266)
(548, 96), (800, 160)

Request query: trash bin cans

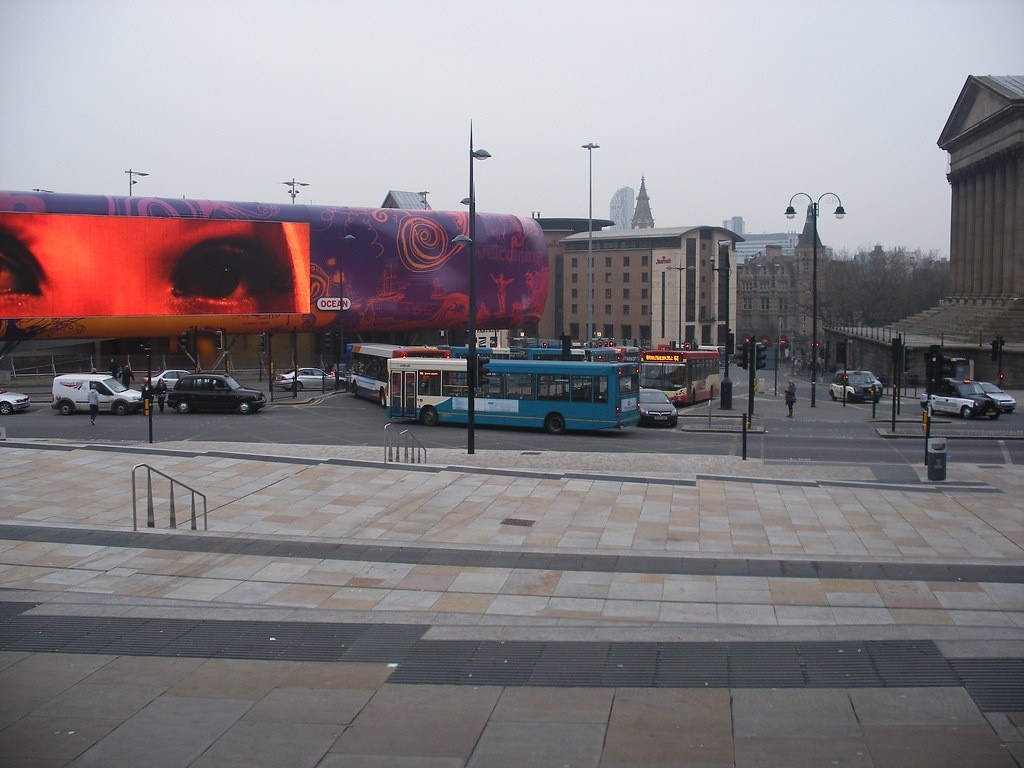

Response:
(759, 379), (765, 393)
(928, 438), (947, 480)
(796, 363), (802, 376)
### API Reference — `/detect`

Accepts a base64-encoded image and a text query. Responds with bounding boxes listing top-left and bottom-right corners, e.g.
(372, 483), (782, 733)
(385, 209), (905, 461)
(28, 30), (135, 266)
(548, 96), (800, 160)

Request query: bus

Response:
(386, 357), (642, 435)
(658, 343), (731, 368)
(641, 350), (720, 405)
(434, 344), (640, 396)
(507, 336), (582, 350)
(343, 342), (451, 408)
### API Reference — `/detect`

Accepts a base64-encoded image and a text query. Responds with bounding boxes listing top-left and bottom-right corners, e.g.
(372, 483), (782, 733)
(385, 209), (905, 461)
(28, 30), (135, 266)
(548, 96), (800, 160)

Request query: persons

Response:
(118, 365), (135, 390)
(998, 377), (1005, 389)
(88, 384), (100, 425)
(92, 368), (98, 374)
(141, 381), (154, 415)
(155, 378), (167, 413)
(784, 380), (797, 418)
(108, 359), (120, 380)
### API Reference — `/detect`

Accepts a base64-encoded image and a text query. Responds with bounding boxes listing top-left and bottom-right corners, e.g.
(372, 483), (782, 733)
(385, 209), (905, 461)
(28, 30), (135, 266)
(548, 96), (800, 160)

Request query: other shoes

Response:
(91, 419), (95, 425)
(786, 414), (792, 417)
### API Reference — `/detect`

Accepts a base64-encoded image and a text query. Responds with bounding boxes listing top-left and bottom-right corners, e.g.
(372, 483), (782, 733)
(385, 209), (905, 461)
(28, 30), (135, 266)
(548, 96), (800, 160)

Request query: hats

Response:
(91, 384), (96, 388)
(789, 381), (795, 384)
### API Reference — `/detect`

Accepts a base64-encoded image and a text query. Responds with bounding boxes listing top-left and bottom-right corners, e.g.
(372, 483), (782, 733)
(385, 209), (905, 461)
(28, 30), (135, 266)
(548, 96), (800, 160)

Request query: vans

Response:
(52, 373), (145, 416)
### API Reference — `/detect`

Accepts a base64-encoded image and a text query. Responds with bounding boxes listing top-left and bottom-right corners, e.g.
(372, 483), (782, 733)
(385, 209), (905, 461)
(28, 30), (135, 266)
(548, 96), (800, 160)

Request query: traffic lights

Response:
(197, 367), (201, 374)
(684, 343), (692, 352)
(143, 398), (149, 416)
(745, 421), (751, 430)
(215, 330), (223, 349)
(609, 340), (614, 347)
(671, 341), (677, 351)
(330, 368), (338, 378)
(737, 336), (750, 370)
(598, 341), (602, 347)
(921, 410), (927, 432)
(604, 341), (608, 347)
(988, 339), (999, 362)
(321, 332), (332, 351)
(182, 326), (193, 354)
(754, 342), (768, 372)
(258, 332), (267, 353)
(754, 378), (759, 386)
(998, 374), (1004, 384)
(692, 340), (698, 351)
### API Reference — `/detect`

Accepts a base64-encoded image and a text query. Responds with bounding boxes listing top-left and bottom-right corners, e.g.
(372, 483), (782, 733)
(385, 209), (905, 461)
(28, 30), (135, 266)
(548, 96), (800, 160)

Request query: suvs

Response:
(830, 369), (876, 404)
(165, 372), (267, 413)
(920, 377), (1000, 420)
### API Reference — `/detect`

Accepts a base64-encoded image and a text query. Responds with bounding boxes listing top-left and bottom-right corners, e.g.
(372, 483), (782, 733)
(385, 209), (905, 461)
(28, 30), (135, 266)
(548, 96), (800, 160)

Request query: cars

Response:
(0, 390), (31, 415)
(143, 368), (217, 388)
(275, 366), (347, 391)
(972, 382), (1016, 412)
(863, 371), (884, 398)
(638, 389), (679, 430)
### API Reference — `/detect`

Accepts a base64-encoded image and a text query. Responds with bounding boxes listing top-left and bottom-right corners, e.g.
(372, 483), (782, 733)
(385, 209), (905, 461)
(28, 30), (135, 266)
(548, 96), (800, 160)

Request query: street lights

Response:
(418, 191), (431, 210)
(334, 233), (357, 391)
(124, 169), (149, 194)
(451, 116), (493, 456)
(667, 256), (696, 350)
(711, 255), (735, 410)
(284, 178), (311, 205)
(783, 193), (848, 408)
(580, 141), (601, 346)
